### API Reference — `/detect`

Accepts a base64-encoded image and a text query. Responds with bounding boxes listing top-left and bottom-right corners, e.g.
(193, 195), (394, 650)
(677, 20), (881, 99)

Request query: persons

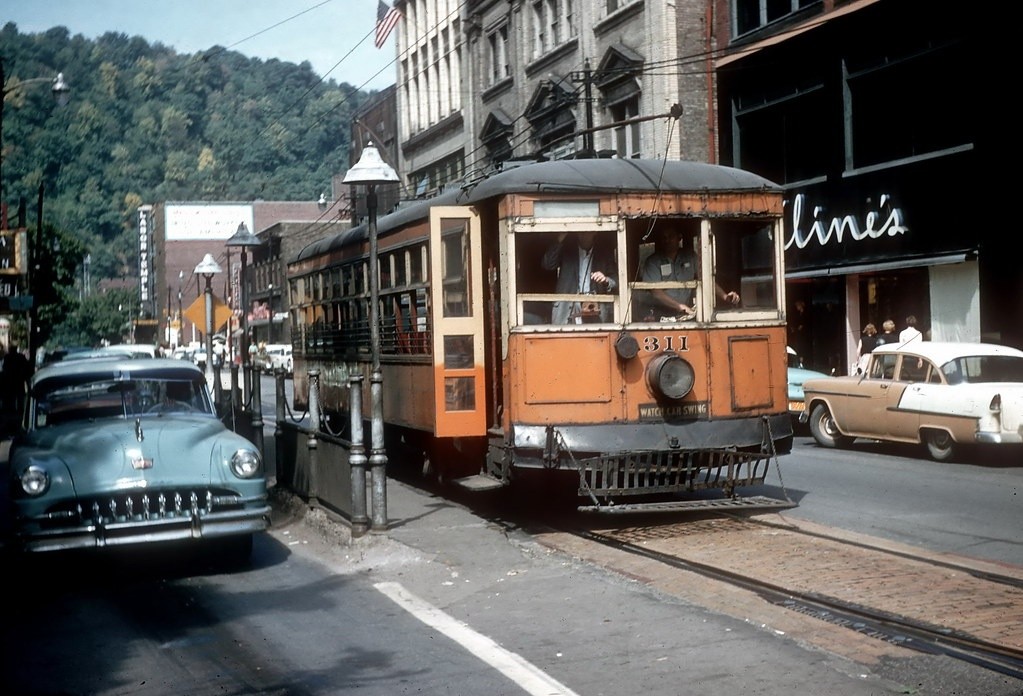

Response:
(248, 340), (258, 366)
(899, 315), (922, 342)
(642, 223), (740, 322)
(856, 323), (879, 375)
(791, 298), (812, 369)
(0, 340), (30, 442)
(541, 232), (619, 324)
(876, 320), (899, 347)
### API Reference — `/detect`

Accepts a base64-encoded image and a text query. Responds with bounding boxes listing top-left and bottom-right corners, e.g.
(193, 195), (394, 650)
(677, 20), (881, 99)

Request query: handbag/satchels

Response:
(798, 362), (804, 369)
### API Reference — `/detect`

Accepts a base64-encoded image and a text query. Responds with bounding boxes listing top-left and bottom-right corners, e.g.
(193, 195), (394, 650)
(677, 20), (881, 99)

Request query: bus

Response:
(284, 158), (796, 514)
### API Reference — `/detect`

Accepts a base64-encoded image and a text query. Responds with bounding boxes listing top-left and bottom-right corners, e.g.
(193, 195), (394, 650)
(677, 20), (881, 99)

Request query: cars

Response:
(10, 359), (272, 571)
(786, 347), (832, 425)
(35, 341), (293, 423)
(803, 340), (1023, 462)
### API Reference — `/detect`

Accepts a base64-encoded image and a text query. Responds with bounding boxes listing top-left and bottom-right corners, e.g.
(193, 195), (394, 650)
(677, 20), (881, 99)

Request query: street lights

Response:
(227, 222), (261, 411)
(341, 142), (402, 533)
(194, 253), (224, 391)
